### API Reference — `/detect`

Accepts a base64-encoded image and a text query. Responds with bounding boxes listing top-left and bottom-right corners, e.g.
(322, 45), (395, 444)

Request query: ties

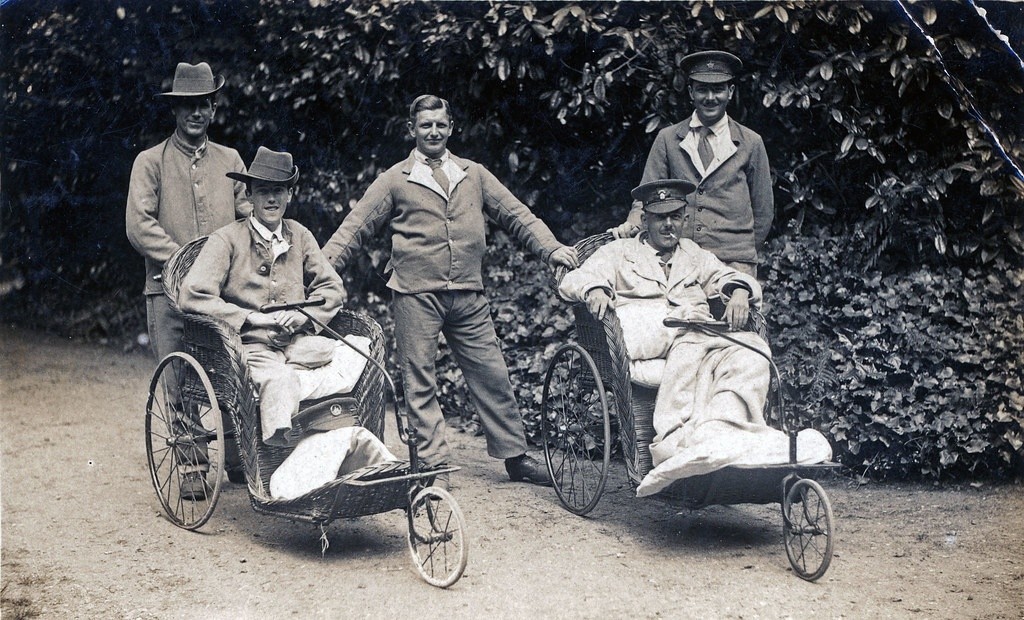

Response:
(698, 127), (714, 170)
(426, 157), (450, 196)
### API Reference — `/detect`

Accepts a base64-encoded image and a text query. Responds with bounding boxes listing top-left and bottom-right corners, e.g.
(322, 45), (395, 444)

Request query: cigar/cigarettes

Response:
(668, 232), (679, 240)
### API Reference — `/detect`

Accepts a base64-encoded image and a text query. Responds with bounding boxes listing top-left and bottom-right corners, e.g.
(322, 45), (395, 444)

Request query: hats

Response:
(153, 62), (225, 100)
(631, 178), (697, 214)
(291, 397), (358, 432)
(226, 146), (300, 187)
(679, 50), (741, 84)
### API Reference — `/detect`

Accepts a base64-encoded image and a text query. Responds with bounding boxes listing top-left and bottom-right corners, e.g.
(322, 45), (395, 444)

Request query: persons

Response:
(125, 62), (348, 501)
(318, 93), (578, 501)
(557, 50), (833, 496)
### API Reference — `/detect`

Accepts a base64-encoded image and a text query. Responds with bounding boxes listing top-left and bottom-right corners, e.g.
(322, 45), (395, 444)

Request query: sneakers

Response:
(228, 472), (246, 485)
(179, 471), (207, 499)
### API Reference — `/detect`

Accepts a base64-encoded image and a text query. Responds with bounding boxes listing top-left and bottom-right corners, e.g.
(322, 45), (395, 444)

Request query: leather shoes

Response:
(425, 469), (449, 499)
(505, 455), (554, 485)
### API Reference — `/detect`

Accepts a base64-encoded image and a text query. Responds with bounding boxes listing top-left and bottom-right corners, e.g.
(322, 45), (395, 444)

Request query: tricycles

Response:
(144, 233), (470, 588)
(542, 229), (844, 583)
